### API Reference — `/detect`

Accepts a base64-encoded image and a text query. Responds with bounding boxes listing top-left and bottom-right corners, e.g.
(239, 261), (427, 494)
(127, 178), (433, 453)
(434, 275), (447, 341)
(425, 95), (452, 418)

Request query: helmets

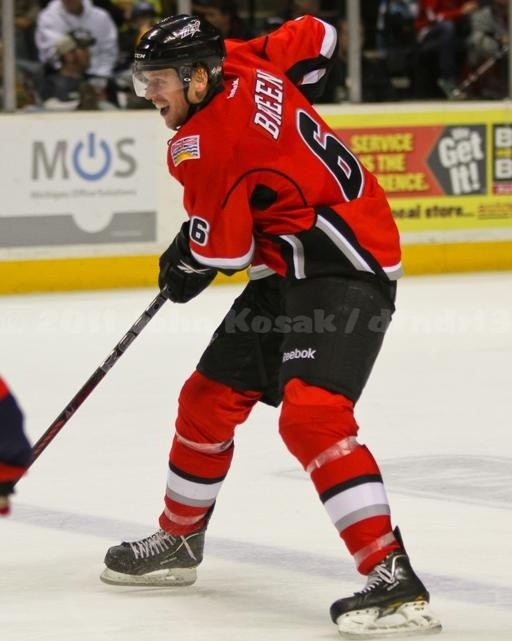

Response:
(132, 15), (226, 103)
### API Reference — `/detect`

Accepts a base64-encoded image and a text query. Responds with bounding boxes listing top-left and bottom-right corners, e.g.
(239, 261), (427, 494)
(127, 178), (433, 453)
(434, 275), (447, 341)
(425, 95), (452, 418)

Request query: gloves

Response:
(158, 221), (216, 303)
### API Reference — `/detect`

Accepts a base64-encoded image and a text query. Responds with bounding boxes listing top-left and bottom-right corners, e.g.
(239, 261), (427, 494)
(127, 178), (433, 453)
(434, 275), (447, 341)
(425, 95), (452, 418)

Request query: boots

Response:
(331, 548), (429, 624)
(105, 530), (205, 575)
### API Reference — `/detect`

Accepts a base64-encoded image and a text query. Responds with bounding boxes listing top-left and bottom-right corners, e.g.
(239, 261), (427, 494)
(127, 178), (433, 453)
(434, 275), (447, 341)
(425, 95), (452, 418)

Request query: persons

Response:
(1, 375), (27, 516)
(1, 0), (511, 110)
(103, 20), (431, 622)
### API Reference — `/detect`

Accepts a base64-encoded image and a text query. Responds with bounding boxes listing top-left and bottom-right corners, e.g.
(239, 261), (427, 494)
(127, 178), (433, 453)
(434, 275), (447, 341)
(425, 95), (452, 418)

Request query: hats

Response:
(57, 30), (96, 57)
